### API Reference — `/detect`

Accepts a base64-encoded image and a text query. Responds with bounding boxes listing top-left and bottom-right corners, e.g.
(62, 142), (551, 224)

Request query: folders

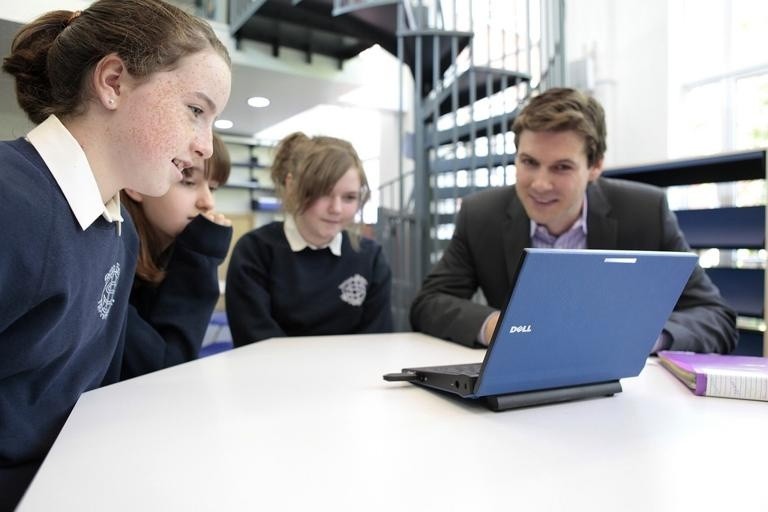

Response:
(655, 347), (767, 401)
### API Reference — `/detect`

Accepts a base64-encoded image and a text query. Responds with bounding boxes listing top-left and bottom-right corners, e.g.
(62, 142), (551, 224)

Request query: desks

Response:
(13, 322), (768, 509)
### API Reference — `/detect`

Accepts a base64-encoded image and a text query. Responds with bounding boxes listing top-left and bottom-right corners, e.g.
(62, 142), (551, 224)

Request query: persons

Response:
(409, 86), (741, 357)
(118, 132), (233, 381)
(224, 131), (393, 348)
(0, 0), (233, 512)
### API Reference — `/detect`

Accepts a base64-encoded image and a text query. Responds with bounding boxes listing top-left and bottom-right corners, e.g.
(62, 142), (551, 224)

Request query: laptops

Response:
(401, 247), (698, 399)
